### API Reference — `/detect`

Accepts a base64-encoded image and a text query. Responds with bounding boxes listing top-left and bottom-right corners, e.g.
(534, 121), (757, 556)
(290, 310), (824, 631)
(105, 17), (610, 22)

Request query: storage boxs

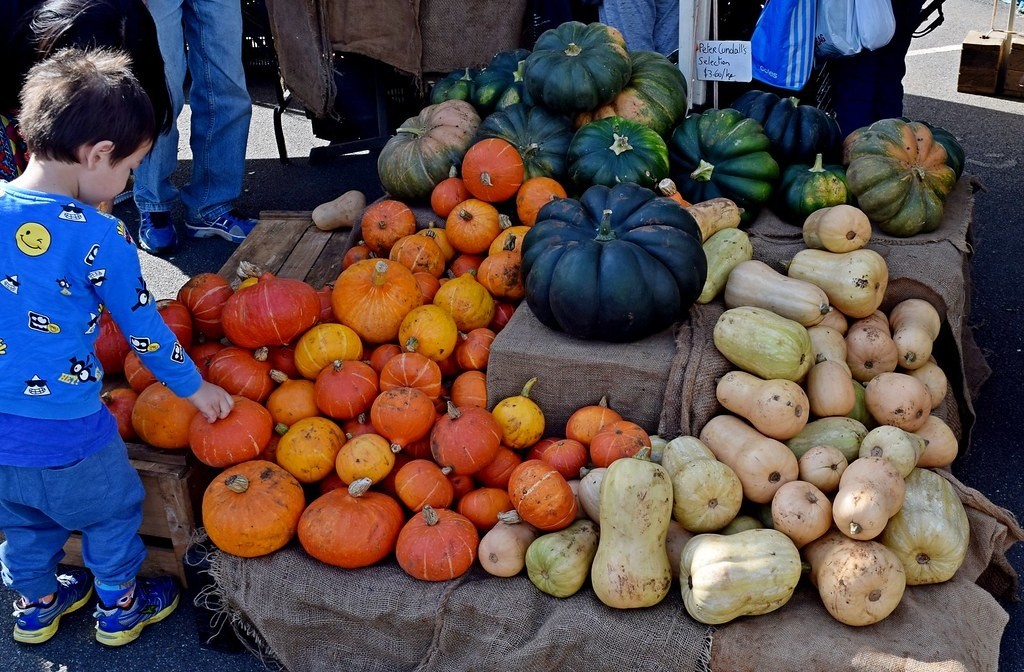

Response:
(217, 210), (353, 291)
(57, 372), (224, 589)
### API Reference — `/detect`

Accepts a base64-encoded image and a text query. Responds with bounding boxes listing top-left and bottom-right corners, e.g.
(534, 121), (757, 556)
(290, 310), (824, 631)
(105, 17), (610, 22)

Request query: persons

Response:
(0, 0), (175, 213)
(0, 47), (234, 646)
(826, 0), (926, 138)
(124, 0), (260, 254)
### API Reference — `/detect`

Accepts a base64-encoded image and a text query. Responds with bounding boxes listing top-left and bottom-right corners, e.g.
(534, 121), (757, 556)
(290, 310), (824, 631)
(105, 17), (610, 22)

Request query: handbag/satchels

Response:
(816, 0), (896, 56)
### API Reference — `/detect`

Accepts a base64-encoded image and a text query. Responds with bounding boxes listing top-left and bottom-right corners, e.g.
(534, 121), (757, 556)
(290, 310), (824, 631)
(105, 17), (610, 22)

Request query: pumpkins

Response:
(94, 20), (972, 627)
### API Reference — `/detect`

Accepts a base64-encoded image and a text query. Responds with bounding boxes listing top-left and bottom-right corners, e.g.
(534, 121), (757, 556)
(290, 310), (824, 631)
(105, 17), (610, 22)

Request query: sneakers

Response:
(93, 576), (182, 645)
(12, 569), (94, 643)
(187, 209), (259, 242)
(138, 210), (179, 253)
